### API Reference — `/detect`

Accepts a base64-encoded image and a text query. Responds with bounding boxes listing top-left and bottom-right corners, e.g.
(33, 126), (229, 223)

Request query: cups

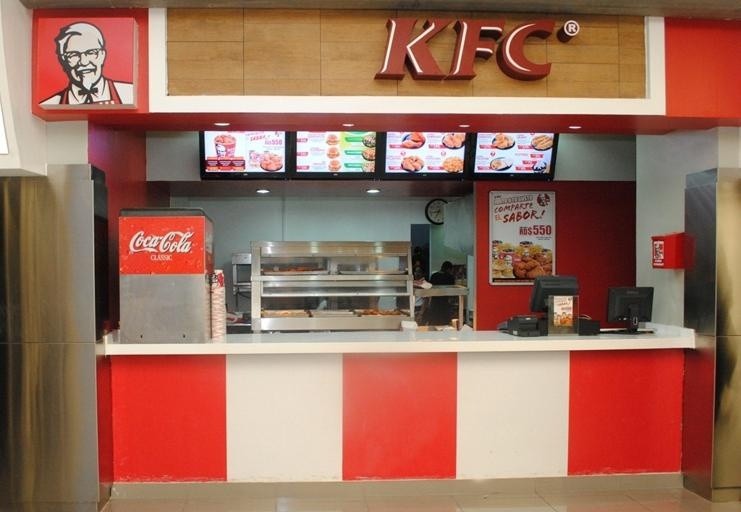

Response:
(211, 270), (225, 338)
(499, 249), (515, 272)
(214, 141), (236, 156)
(451, 317), (458, 329)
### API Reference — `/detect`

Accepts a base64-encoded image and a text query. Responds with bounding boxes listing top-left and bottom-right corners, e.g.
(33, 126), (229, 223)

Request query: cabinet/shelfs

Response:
(248, 241), (414, 333)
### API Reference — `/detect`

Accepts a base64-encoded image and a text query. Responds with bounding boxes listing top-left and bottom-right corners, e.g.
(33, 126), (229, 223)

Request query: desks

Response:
(416, 283), (469, 331)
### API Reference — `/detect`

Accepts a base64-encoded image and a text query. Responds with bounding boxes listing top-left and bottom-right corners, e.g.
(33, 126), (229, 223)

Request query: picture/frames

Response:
(489, 191), (557, 286)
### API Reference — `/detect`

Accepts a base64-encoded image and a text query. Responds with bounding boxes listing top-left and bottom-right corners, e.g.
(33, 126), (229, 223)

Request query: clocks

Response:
(425, 199), (449, 225)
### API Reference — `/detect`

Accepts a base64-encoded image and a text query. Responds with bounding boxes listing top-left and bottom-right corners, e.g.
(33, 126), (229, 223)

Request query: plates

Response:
(400, 133), (426, 149)
(260, 161), (282, 170)
(532, 137), (553, 151)
(442, 134), (464, 149)
(402, 158), (424, 171)
(491, 158), (513, 170)
(363, 134), (376, 172)
(492, 138), (514, 149)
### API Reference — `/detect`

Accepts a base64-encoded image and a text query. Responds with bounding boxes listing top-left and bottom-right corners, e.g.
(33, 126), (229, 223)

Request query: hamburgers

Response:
(327, 135), (338, 146)
(329, 160), (341, 170)
(327, 148), (338, 158)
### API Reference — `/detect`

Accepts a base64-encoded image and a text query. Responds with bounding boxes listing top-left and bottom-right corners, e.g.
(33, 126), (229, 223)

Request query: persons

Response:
(430, 261), (456, 325)
(40, 22), (133, 105)
(414, 262), (432, 315)
(653, 243), (663, 260)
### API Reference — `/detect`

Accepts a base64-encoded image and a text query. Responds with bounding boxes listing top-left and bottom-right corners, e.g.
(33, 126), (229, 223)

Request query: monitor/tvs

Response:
(529, 276), (578, 312)
(608, 287), (654, 322)
